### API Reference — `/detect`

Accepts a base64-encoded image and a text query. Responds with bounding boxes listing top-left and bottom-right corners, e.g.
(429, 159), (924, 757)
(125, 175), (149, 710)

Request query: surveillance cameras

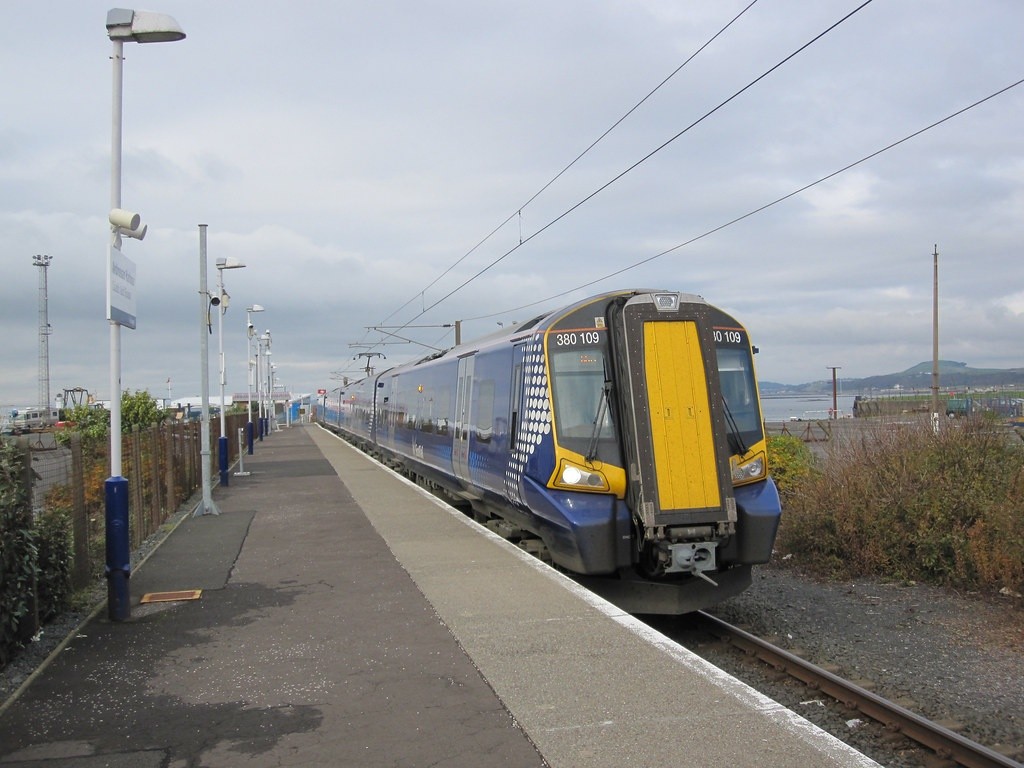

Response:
(209, 292), (220, 306)
(248, 323), (253, 328)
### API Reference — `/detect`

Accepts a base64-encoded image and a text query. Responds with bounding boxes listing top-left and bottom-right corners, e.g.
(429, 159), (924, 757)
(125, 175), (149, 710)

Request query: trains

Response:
(315, 290), (781, 617)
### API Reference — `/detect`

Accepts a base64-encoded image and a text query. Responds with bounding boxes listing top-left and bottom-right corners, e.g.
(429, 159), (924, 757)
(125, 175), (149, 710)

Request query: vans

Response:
(946, 399), (989, 420)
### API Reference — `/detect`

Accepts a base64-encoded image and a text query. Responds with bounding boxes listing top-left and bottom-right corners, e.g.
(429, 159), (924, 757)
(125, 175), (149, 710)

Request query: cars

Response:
(985, 398), (1023, 414)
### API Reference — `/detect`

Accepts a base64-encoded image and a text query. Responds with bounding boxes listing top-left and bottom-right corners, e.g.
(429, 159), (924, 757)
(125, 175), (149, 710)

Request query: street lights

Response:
(246, 304), (265, 456)
(215, 258), (246, 487)
(256, 335), (270, 441)
(265, 350), (272, 437)
(103, 7), (186, 622)
